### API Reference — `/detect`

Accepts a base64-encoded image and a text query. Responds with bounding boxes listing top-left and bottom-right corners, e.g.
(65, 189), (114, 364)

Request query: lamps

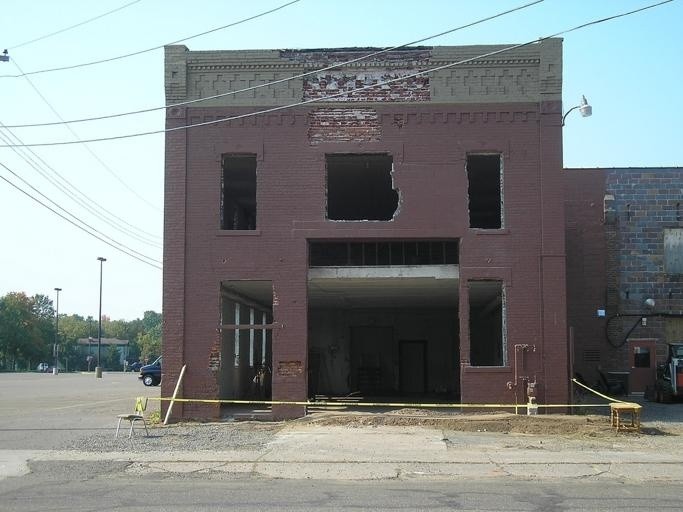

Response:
(560, 95), (592, 126)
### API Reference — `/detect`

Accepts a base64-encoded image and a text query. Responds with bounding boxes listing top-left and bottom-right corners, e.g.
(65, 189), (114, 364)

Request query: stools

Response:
(608, 401), (642, 434)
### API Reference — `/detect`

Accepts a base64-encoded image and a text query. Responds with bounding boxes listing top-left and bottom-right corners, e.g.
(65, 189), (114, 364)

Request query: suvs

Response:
(137, 355), (161, 386)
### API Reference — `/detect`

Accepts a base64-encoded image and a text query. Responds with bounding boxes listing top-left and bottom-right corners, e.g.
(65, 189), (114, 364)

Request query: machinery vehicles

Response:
(643, 343), (683, 403)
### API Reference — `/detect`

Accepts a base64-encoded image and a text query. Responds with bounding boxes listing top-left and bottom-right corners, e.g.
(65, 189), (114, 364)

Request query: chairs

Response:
(114, 395), (148, 439)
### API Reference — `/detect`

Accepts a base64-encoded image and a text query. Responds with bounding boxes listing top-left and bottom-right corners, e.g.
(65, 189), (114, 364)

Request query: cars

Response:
(127, 362), (141, 371)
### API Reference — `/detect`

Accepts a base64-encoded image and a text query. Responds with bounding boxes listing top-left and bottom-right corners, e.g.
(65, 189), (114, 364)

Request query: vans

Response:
(37, 362), (47, 371)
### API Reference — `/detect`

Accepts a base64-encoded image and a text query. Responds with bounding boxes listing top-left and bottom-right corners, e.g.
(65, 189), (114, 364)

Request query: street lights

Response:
(94, 257), (106, 378)
(54, 288), (62, 374)
